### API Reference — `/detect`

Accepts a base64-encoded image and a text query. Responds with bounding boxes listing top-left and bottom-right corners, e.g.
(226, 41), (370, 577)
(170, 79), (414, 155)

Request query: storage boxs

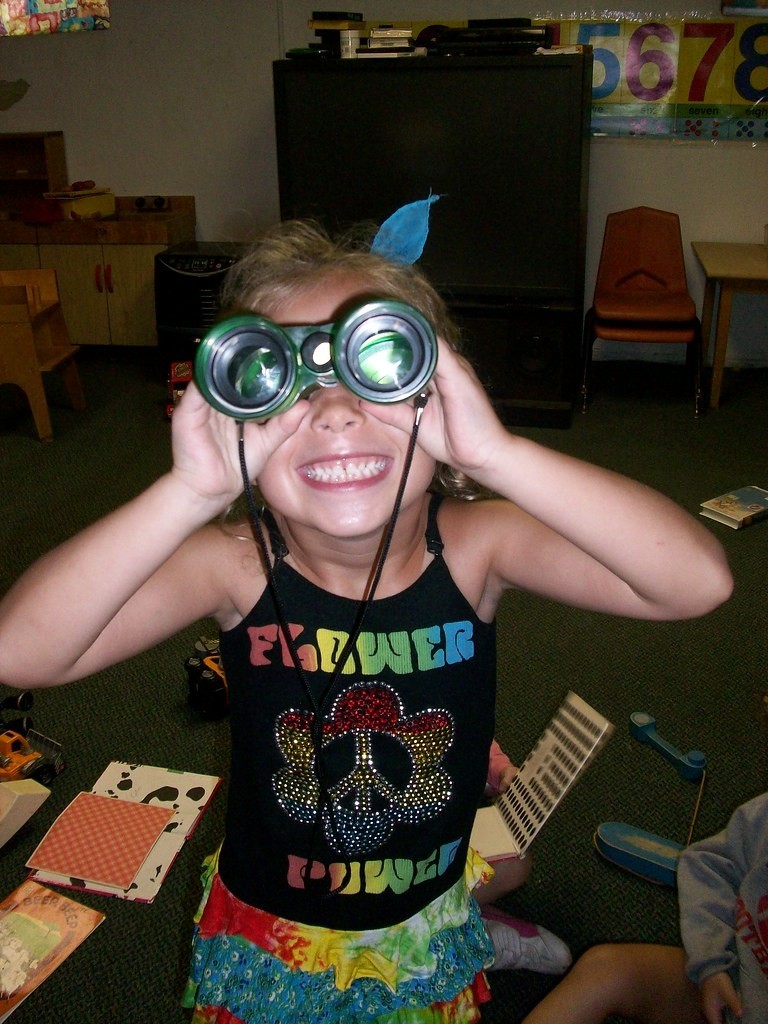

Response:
(0, 168), (68, 195)
(0, 130), (69, 177)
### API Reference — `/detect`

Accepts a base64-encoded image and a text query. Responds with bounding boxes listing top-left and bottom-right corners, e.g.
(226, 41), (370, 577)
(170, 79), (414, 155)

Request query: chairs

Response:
(579, 325), (705, 423)
(579, 204), (705, 399)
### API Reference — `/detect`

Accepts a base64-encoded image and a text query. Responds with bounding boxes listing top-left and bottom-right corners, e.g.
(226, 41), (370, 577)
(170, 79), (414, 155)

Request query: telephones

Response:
(629, 711), (705, 783)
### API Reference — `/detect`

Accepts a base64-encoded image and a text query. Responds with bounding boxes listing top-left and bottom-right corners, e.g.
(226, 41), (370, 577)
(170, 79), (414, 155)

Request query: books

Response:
(699, 485), (768, 529)
(469, 690), (614, 863)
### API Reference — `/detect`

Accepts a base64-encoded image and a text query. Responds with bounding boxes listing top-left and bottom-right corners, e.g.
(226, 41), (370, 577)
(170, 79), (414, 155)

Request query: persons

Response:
(470, 740), (572, 974)
(0, 218), (734, 1024)
(524, 793), (768, 1024)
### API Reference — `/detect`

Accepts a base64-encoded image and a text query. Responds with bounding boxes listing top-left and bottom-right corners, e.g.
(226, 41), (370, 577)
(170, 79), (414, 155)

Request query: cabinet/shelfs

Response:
(0, 267), (87, 444)
(272, 45), (598, 435)
(0, 196), (196, 348)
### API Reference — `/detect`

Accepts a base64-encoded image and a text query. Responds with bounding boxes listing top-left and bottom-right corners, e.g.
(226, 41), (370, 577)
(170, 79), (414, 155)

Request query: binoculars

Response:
(194, 293), (437, 426)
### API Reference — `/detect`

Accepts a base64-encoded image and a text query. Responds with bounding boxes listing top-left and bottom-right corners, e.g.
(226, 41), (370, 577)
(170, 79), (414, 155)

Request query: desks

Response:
(689, 239), (768, 412)
